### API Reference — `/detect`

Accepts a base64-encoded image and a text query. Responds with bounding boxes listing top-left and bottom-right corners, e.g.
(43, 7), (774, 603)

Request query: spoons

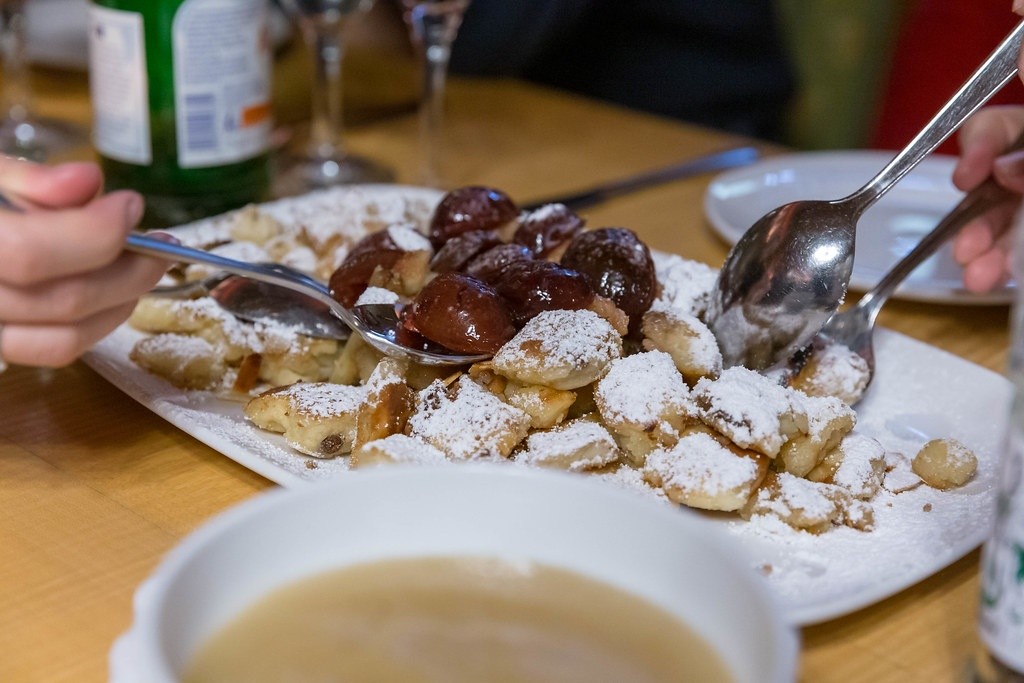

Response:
(0, 188), (493, 363)
(518, 142), (760, 226)
(700, 18), (1024, 373)
(774, 133), (1024, 418)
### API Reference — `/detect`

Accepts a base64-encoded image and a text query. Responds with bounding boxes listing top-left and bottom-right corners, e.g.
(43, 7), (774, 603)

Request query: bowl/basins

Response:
(106, 461), (801, 683)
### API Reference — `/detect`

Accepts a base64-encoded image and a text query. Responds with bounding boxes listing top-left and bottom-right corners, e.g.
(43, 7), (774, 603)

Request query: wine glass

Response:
(391, 3), (481, 164)
(266, 0), (392, 199)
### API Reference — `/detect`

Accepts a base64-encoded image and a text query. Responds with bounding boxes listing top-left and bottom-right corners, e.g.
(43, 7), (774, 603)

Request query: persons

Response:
(953, 0), (1024, 296)
(0, 150), (184, 372)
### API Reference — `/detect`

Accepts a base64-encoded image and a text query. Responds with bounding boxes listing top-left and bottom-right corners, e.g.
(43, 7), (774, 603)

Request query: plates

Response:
(698, 148), (1024, 312)
(75, 182), (1017, 629)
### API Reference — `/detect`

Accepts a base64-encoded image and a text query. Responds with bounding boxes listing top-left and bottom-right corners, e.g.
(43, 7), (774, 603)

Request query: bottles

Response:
(86, 0), (275, 229)
